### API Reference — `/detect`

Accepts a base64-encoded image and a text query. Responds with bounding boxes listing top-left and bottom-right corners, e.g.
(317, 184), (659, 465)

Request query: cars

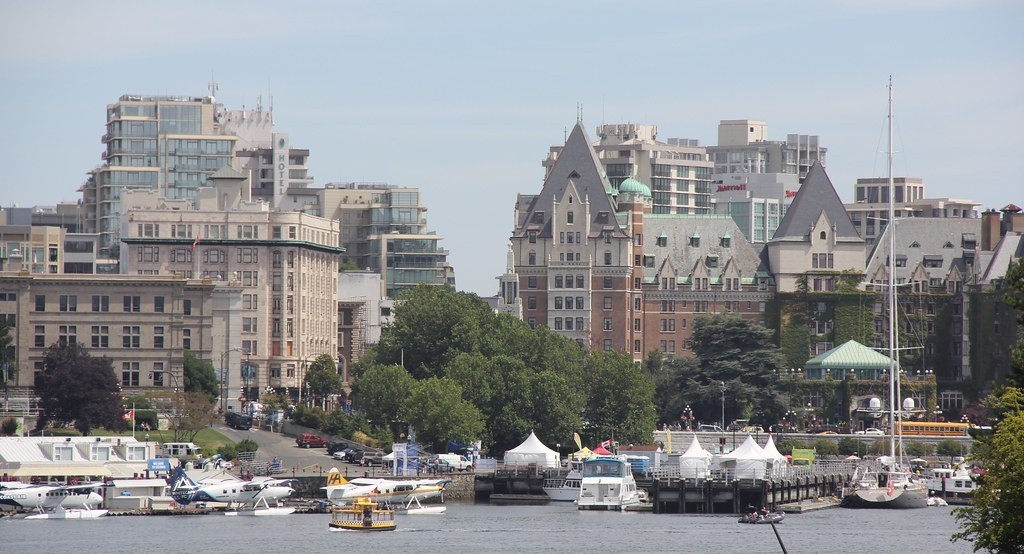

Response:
(770, 425), (798, 433)
(332, 447), (363, 460)
(806, 425), (841, 433)
(855, 427), (886, 435)
(561, 457), (584, 467)
(727, 425), (741, 431)
(347, 448), (367, 463)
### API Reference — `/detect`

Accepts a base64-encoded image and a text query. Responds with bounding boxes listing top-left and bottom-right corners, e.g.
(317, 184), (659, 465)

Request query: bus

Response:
(163, 443), (203, 459)
(894, 421), (976, 435)
(0, 398), (60, 414)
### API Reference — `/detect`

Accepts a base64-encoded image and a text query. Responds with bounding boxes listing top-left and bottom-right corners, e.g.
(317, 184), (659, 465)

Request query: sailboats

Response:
(843, 72), (929, 508)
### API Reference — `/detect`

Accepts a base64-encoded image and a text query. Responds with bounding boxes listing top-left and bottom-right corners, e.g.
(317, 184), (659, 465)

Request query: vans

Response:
(432, 453), (472, 472)
(226, 412), (253, 430)
(700, 425), (722, 432)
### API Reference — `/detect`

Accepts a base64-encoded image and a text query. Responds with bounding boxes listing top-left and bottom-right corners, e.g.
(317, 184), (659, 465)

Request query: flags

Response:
(192, 233), (200, 250)
(574, 432), (581, 450)
(122, 411), (133, 422)
(602, 439), (612, 448)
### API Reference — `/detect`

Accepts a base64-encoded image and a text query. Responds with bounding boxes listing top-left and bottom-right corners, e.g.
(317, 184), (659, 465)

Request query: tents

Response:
(504, 429), (561, 469)
(568, 446), (598, 460)
(678, 433), (787, 479)
(592, 446), (613, 455)
(805, 340), (898, 380)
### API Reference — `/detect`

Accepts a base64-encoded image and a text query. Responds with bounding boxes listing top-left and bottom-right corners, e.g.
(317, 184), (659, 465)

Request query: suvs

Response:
(296, 433), (329, 448)
(355, 451), (388, 467)
(327, 441), (348, 455)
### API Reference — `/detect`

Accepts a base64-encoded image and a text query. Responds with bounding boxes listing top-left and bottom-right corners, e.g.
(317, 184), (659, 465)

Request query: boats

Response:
(915, 469), (983, 505)
(738, 511), (786, 524)
(575, 456), (647, 511)
(926, 496), (948, 507)
(542, 469), (582, 501)
(328, 497), (397, 531)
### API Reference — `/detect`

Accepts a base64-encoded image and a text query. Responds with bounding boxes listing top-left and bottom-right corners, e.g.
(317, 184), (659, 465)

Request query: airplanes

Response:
(0, 481), (116, 519)
(169, 466), (299, 516)
(320, 466), (453, 514)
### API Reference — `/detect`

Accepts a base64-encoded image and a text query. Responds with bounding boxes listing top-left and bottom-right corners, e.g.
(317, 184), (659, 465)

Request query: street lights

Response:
(756, 410), (766, 433)
(684, 404), (692, 431)
(305, 353), (323, 408)
(933, 405), (944, 422)
(220, 348), (242, 414)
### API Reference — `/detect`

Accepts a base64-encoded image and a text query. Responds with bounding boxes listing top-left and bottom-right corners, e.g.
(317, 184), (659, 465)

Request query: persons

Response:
(752, 507), (769, 519)
(140, 421), (150, 431)
(198, 455), (204, 469)
